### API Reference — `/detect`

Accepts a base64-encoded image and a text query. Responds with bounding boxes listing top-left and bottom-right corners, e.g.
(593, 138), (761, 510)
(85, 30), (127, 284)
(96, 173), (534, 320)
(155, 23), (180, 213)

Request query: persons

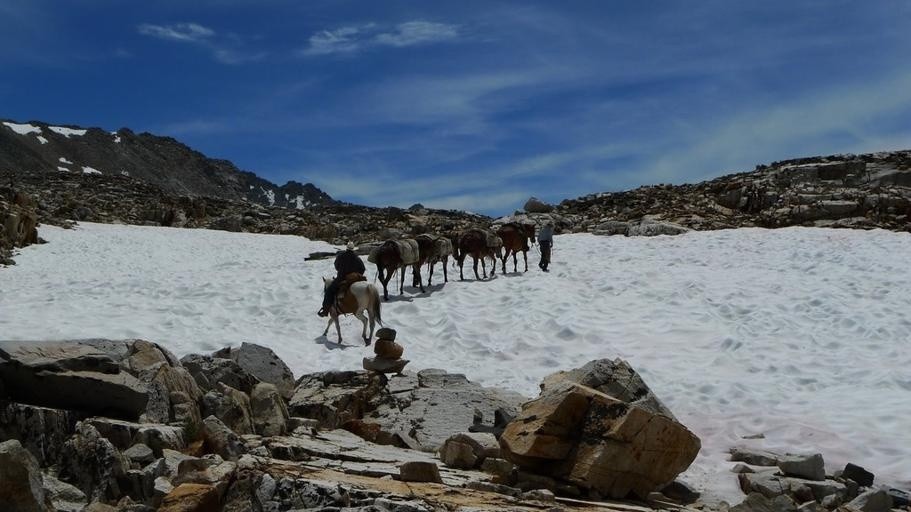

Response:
(536, 217), (553, 273)
(315, 240), (366, 317)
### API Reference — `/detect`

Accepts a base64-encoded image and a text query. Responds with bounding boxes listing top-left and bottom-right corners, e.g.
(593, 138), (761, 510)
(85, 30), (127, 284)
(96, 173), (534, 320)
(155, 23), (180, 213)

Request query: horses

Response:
(374, 221), (537, 302)
(322, 276), (384, 346)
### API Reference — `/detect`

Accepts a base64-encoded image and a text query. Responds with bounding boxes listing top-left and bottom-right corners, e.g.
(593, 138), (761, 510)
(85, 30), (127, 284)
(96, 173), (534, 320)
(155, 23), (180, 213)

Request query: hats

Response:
(343, 242), (360, 251)
(547, 221), (554, 227)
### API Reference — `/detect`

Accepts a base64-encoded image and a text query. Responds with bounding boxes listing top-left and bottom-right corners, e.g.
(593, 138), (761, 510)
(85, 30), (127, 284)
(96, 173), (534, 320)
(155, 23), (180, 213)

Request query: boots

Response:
(320, 304), (329, 317)
(538, 261), (549, 271)
